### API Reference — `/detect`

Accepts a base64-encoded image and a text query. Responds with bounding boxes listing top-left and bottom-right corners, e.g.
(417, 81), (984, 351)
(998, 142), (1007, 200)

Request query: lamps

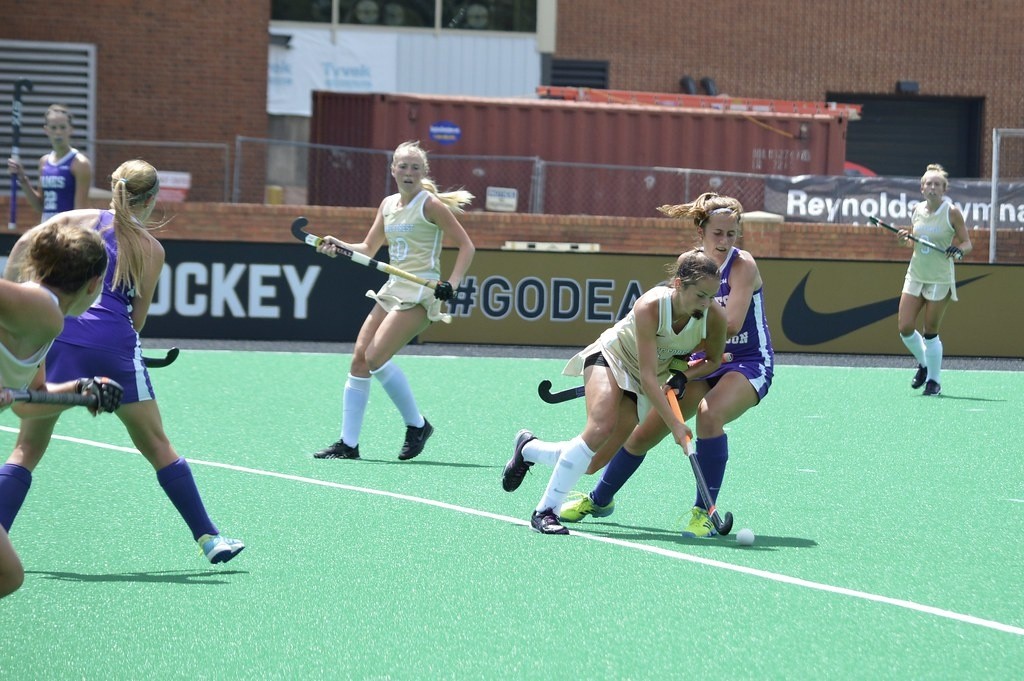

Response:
(897, 79), (919, 92)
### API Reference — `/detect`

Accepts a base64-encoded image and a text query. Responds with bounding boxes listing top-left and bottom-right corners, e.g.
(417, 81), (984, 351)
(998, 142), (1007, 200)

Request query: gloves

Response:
(434, 282), (454, 302)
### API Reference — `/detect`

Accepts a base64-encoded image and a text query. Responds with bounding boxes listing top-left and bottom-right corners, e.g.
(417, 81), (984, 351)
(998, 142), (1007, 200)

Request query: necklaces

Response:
(672, 314), (690, 323)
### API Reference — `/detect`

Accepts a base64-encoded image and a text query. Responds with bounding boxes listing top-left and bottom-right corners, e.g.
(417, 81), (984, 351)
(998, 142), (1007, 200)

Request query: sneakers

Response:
(314, 439), (361, 460)
(922, 379), (942, 395)
(676, 506), (717, 538)
(398, 416), (434, 460)
(558, 491), (615, 522)
(501, 429), (538, 492)
(197, 533), (245, 564)
(530, 506), (569, 534)
(911, 364), (927, 389)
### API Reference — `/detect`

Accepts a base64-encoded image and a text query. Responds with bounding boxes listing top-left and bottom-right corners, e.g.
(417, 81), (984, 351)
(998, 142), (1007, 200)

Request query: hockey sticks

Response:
(868, 215), (963, 260)
(537, 351), (734, 406)
(143, 343), (181, 368)
(291, 218), (458, 299)
(0, 386), (98, 407)
(666, 388), (734, 536)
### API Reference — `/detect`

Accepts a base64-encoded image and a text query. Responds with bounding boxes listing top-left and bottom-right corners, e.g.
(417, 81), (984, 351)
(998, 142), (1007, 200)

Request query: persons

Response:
(314, 142), (475, 460)
(896, 165), (972, 395)
(7, 104), (91, 224)
(561, 192), (774, 537)
(502, 248), (728, 535)
(0, 157), (245, 563)
(0, 224), (125, 599)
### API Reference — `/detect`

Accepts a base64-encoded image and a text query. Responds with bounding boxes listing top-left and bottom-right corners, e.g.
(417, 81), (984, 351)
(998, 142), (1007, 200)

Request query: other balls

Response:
(737, 528), (755, 546)
